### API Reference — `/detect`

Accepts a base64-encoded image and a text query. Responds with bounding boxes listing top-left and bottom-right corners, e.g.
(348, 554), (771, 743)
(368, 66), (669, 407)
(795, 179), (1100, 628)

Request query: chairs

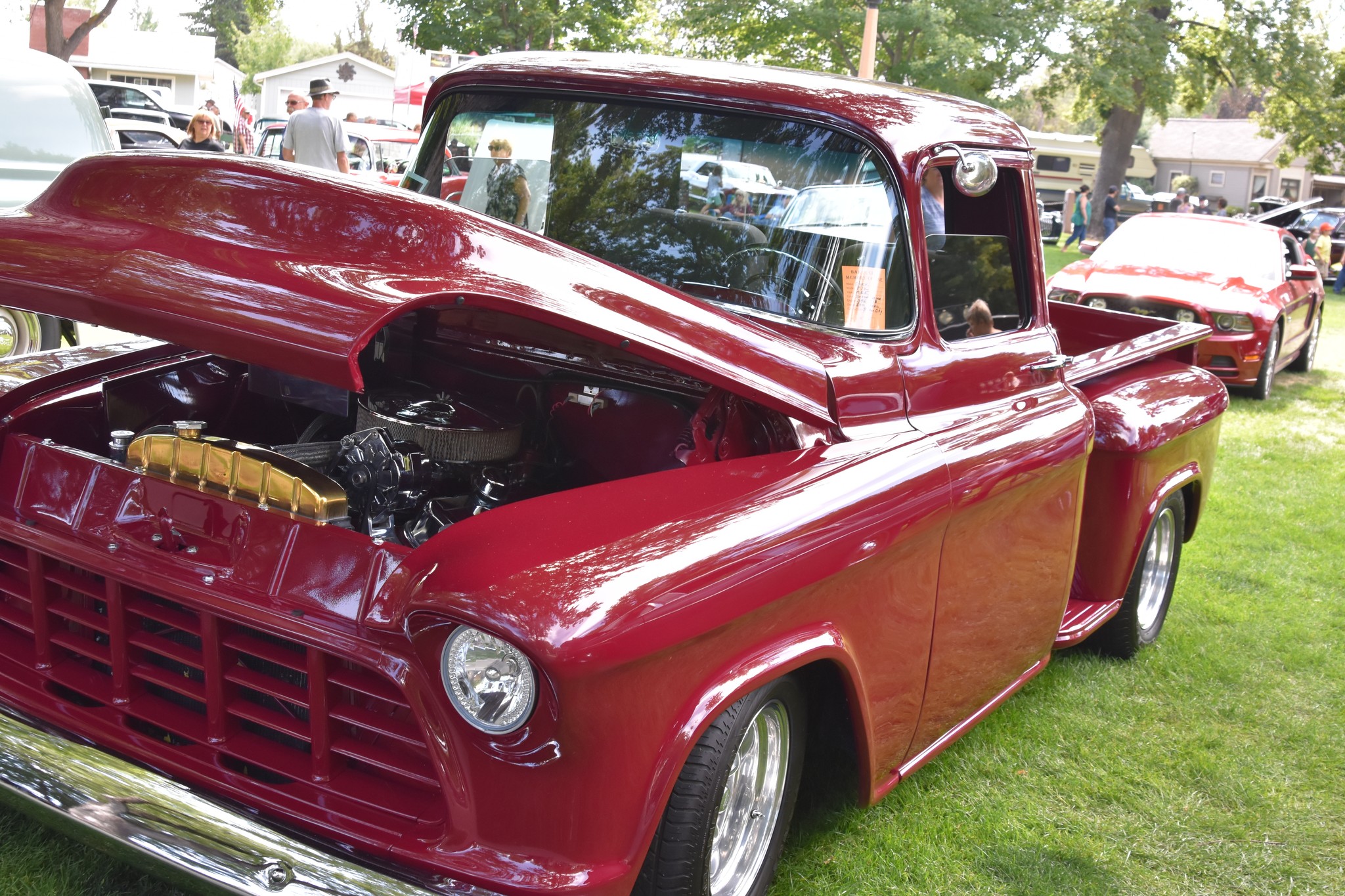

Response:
(823, 241), (979, 328)
(633, 206), (772, 293)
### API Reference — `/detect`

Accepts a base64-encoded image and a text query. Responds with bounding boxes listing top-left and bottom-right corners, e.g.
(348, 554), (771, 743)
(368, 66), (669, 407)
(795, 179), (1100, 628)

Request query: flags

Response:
(233, 80), (246, 128)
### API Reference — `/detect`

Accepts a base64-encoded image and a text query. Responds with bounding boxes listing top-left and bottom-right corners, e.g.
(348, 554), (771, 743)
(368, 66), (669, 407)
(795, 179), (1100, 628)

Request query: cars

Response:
(84, 77), (470, 206)
(1115, 180), (1345, 284)
(1046, 211), (1325, 400)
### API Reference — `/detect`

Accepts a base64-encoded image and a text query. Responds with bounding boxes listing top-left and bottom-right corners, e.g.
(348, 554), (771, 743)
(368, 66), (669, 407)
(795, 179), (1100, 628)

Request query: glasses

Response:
(328, 93), (336, 99)
(197, 119), (212, 125)
(488, 146), (502, 151)
(285, 101), (305, 105)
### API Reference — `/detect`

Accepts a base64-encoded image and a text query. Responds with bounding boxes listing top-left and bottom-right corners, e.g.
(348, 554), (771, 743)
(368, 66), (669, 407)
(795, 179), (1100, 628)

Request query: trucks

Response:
(1026, 129), (1159, 213)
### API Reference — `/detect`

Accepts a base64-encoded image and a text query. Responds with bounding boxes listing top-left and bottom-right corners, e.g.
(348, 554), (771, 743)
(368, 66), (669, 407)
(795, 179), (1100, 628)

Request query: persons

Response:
(282, 79), (351, 174)
(233, 108), (254, 156)
(484, 137), (531, 230)
(1062, 185), (1092, 254)
(920, 166), (945, 254)
(446, 140), (469, 172)
(1169, 187), (1227, 217)
(1301, 222), (1345, 294)
(279, 89), (310, 162)
(178, 97), (224, 153)
(964, 299), (1003, 338)
(699, 164), (792, 233)
(1103, 184), (1120, 240)
(345, 112), (421, 172)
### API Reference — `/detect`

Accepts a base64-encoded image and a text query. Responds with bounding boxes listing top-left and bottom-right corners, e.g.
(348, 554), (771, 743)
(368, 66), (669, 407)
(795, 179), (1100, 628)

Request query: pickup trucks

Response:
(0, 47), (1231, 896)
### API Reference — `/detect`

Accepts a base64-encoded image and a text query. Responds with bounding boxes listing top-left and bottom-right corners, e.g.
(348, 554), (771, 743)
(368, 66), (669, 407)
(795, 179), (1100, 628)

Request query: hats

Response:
(450, 139), (458, 144)
(242, 109), (250, 113)
(1320, 223), (1335, 232)
(1178, 187), (1186, 196)
(306, 78), (340, 96)
(1199, 195), (1206, 200)
(1110, 185), (1119, 193)
